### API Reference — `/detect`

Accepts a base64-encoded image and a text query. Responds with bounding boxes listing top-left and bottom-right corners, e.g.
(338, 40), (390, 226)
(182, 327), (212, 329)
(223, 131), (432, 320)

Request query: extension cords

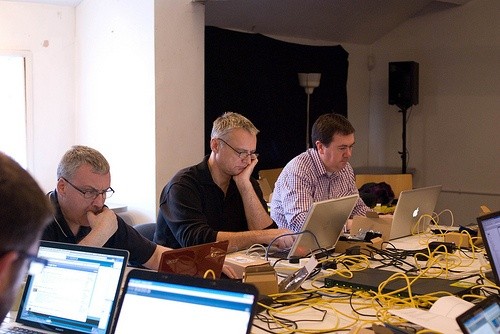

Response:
(259, 257), (335, 282)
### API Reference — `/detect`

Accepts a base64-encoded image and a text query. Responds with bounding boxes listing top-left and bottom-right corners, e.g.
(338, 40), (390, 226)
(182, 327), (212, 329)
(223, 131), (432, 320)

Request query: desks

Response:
(247, 225), (500, 334)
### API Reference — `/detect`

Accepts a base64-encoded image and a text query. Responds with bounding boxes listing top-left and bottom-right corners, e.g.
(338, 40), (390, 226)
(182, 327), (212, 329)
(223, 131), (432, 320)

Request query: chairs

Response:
(355, 175), (412, 200)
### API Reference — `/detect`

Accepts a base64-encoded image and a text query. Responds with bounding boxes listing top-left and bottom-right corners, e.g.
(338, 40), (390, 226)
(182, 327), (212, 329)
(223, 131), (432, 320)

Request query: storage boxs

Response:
(226, 254), (278, 298)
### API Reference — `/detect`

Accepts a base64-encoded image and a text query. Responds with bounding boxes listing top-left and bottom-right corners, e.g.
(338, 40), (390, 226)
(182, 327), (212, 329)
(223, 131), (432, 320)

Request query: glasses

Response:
(0, 250), (47, 276)
(58, 177), (114, 198)
(218, 138), (259, 160)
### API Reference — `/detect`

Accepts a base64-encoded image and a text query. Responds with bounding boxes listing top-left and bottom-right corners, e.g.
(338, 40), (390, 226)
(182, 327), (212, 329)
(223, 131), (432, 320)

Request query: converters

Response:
(346, 245), (360, 255)
(428, 241), (456, 253)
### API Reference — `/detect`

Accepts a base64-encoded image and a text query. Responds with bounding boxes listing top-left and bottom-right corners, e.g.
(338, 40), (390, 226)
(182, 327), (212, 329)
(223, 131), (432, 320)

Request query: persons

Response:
(0, 151), (55, 327)
(269, 114), (394, 233)
(41, 145), (239, 279)
(151, 113), (296, 254)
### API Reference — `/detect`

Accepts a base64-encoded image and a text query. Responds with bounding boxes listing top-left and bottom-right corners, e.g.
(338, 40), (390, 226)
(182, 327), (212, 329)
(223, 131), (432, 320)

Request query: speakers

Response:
(388, 61), (420, 105)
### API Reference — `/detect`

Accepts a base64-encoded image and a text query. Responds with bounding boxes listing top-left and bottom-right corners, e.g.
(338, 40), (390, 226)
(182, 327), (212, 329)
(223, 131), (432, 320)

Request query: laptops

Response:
(388, 184), (442, 241)
(110, 268), (259, 334)
(455, 293), (500, 334)
(0, 239), (130, 334)
(477, 211), (500, 288)
(239, 194), (359, 260)
(158, 239), (229, 280)
